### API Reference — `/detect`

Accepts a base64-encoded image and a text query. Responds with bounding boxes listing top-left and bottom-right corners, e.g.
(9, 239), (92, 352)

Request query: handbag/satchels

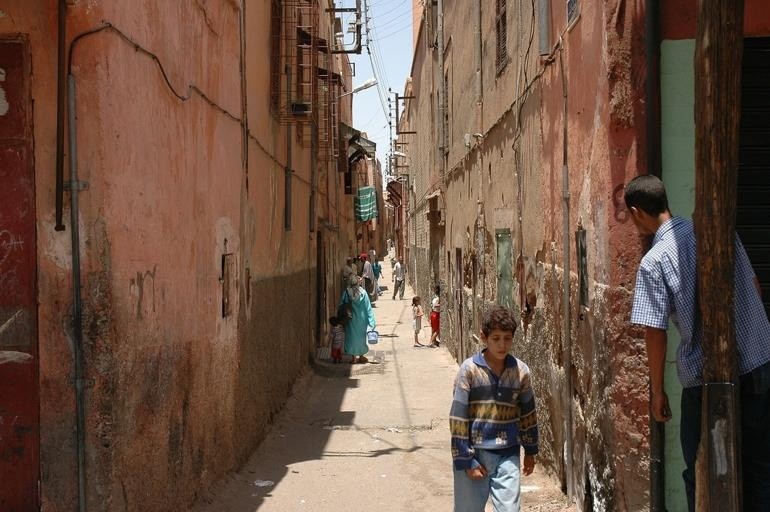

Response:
(337, 303), (352, 321)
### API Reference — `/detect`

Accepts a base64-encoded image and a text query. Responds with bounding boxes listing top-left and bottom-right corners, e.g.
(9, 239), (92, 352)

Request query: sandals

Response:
(351, 356), (367, 363)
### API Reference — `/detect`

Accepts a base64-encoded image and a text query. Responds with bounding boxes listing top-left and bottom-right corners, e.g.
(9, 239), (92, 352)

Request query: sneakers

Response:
(392, 296), (403, 300)
(426, 343), (437, 348)
(414, 342), (425, 347)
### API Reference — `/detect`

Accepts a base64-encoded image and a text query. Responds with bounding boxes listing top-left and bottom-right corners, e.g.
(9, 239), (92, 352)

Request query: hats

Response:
(359, 254), (368, 259)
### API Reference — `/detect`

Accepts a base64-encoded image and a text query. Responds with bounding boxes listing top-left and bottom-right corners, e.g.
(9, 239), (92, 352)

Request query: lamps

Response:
(336, 78), (379, 99)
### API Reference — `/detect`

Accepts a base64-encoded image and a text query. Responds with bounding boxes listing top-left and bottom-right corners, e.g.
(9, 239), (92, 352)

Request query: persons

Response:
(623, 173), (770, 512)
(428, 285), (440, 347)
(327, 315), (348, 364)
(338, 273), (376, 364)
(449, 307), (538, 512)
(342, 254), (384, 308)
(412, 297), (426, 347)
(392, 255), (407, 300)
(369, 246), (376, 263)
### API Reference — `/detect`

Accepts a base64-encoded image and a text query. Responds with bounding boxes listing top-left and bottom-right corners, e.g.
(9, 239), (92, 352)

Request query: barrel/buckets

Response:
(367, 331), (378, 344)
